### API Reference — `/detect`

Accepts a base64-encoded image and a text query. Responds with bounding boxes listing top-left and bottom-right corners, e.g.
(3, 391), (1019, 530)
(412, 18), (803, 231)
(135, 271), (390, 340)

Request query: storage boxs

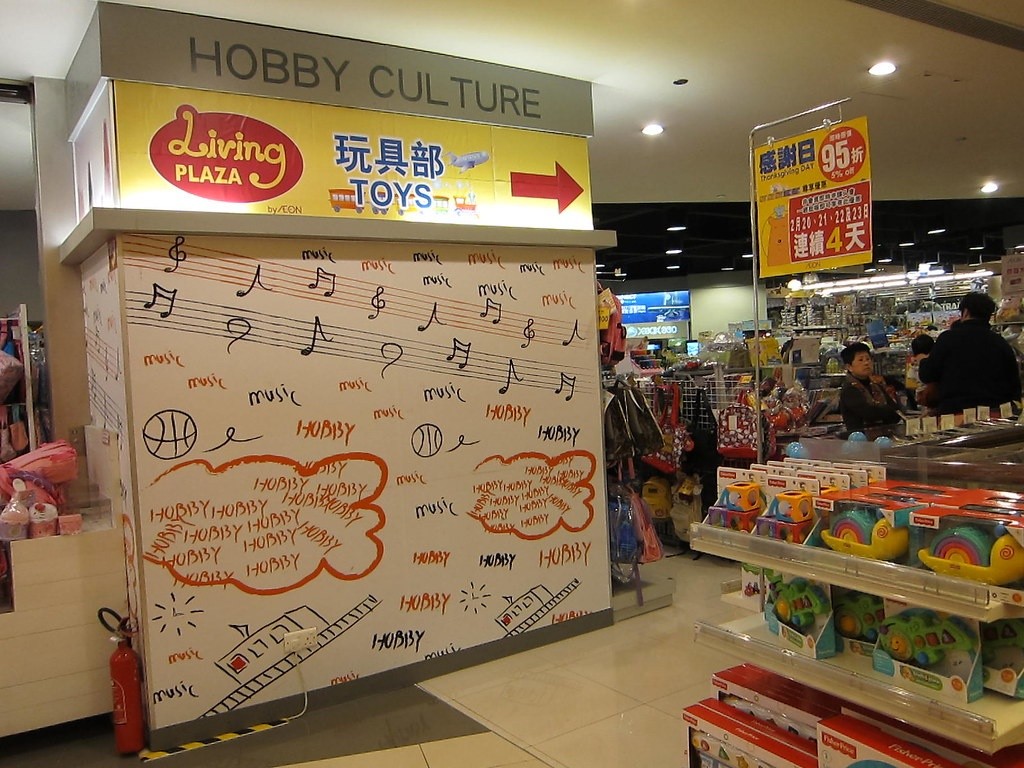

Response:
(742, 320), (774, 331)
(681, 480), (1024, 768)
(708, 343), (744, 368)
(781, 294), (961, 336)
(57, 513), (84, 536)
(775, 336), (821, 364)
(745, 339), (782, 366)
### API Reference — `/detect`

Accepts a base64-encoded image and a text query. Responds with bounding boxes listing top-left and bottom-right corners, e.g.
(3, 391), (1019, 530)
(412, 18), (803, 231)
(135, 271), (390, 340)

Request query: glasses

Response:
(957, 310), (962, 317)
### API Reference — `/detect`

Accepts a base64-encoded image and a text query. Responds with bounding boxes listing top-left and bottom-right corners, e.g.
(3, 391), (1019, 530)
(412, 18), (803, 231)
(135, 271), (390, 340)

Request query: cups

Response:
(58, 514), (82, 535)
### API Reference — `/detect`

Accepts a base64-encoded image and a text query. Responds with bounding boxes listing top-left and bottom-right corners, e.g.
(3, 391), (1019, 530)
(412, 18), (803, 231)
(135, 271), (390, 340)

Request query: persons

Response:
(839, 343), (905, 430)
(910, 290), (1022, 417)
(905, 334), (935, 411)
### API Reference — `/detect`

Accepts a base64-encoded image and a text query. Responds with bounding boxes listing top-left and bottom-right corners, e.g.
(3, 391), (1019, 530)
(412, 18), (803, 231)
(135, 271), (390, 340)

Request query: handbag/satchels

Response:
(0, 405), (28, 462)
(681, 389), (722, 476)
(0, 349), (23, 403)
(641, 385), (694, 474)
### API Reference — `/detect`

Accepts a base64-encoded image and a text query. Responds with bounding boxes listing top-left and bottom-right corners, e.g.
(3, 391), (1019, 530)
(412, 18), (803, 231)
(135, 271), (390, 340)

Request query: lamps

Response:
(802, 271), (821, 285)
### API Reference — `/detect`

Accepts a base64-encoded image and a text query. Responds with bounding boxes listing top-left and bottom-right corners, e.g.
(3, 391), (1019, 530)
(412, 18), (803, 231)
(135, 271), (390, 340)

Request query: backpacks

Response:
(597, 279), (627, 367)
(670, 479), (702, 542)
(717, 390), (758, 459)
(761, 409), (777, 460)
(608, 378), (665, 457)
(641, 476), (672, 519)
(608, 481), (662, 564)
(600, 381), (635, 462)
(1, 439), (77, 495)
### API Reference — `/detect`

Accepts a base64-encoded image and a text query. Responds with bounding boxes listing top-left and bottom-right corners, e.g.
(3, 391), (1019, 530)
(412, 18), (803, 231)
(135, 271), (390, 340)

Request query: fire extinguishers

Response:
(98, 607), (144, 755)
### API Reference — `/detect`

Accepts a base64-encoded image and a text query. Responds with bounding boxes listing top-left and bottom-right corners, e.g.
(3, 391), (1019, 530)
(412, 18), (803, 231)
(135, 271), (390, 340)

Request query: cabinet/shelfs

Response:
(0, 303), (37, 454)
(0, 423), (127, 738)
(686, 521), (1024, 753)
